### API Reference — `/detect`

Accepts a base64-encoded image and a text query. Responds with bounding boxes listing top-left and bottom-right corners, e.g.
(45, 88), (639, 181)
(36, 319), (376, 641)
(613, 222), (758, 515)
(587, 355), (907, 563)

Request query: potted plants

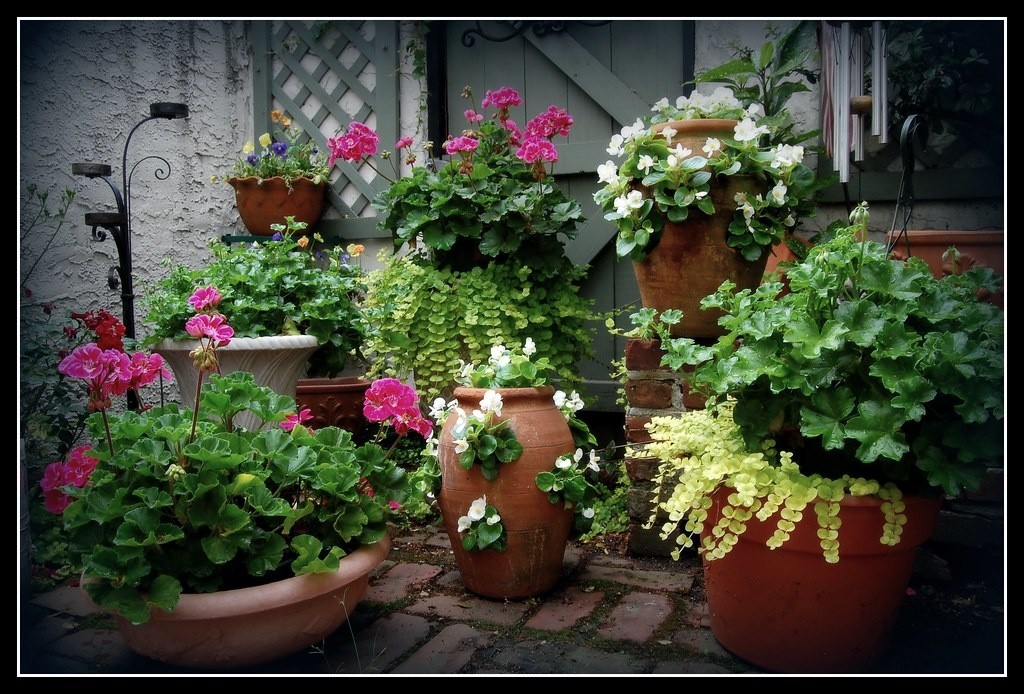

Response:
(360, 246), (643, 437)
(608, 201), (1015, 673)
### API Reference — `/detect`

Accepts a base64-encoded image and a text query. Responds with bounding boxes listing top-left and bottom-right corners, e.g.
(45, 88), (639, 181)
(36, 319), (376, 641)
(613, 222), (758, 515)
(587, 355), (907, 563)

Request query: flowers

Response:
(592, 85), (821, 262)
(414, 337), (600, 552)
(325, 85), (591, 257)
(39, 285), (441, 624)
(139, 215), (369, 379)
(208, 110), (379, 196)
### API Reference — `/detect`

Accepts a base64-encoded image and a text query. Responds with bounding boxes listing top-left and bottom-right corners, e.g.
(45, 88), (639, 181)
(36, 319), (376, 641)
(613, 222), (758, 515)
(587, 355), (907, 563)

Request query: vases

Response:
(622, 119), (776, 335)
(145, 336), (317, 436)
(425, 239), (496, 269)
(435, 387), (577, 599)
(79, 531), (392, 663)
(885, 227), (1004, 304)
